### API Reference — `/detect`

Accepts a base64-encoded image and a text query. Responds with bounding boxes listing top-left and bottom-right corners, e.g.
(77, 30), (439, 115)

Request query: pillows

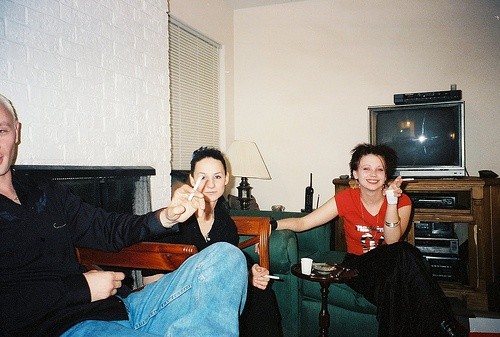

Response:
(228, 193), (261, 211)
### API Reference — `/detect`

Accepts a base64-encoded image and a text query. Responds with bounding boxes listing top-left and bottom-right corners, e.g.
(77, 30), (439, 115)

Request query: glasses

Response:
(192, 146), (215, 158)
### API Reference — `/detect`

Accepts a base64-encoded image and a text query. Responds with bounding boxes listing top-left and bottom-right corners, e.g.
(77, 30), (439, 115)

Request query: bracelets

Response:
(270, 219), (278, 231)
(383, 220), (401, 228)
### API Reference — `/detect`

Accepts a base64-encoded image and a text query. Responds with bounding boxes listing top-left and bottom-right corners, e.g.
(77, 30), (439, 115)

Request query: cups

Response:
(300, 257), (313, 275)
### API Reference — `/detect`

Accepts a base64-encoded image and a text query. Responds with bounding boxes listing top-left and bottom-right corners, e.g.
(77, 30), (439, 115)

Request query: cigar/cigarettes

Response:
(262, 273), (280, 280)
(188, 175), (203, 202)
(394, 177), (415, 182)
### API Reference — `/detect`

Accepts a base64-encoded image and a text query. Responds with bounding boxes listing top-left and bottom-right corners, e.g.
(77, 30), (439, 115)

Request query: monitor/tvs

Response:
(368, 100), (466, 180)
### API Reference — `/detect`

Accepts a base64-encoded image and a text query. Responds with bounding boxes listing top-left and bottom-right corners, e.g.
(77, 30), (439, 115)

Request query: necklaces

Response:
(360, 202), (381, 240)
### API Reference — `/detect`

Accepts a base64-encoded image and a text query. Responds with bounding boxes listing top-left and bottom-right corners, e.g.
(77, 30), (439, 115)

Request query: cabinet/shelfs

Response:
(332, 178), (500, 313)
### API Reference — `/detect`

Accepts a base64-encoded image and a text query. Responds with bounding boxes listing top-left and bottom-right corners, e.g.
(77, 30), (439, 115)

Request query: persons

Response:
(141, 147), (284, 337)
(255, 144), (457, 337)
(0, 93), (247, 337)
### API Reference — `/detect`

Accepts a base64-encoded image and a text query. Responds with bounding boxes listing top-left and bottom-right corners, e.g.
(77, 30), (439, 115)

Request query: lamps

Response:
(223, 139), (271, 209)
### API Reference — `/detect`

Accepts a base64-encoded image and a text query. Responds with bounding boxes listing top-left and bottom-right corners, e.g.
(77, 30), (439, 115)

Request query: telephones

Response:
(301, 173), (313, 213)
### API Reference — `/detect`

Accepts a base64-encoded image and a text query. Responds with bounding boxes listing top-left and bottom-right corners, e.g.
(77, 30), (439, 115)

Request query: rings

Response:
(399, 190), (402, 193)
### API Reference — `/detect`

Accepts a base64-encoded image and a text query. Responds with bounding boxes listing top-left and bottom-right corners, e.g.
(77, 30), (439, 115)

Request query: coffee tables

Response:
(291, 263), (368, 337)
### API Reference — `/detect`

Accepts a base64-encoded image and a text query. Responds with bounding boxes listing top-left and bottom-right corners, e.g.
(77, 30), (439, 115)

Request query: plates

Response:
(313, 265), (339, 275)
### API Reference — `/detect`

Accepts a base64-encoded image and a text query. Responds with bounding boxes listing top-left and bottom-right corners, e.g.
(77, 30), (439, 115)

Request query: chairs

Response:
(11, 164), (272, 285)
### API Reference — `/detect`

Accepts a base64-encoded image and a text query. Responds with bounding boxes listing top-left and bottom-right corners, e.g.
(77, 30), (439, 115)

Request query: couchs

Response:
(229, 209), (381, 337)
